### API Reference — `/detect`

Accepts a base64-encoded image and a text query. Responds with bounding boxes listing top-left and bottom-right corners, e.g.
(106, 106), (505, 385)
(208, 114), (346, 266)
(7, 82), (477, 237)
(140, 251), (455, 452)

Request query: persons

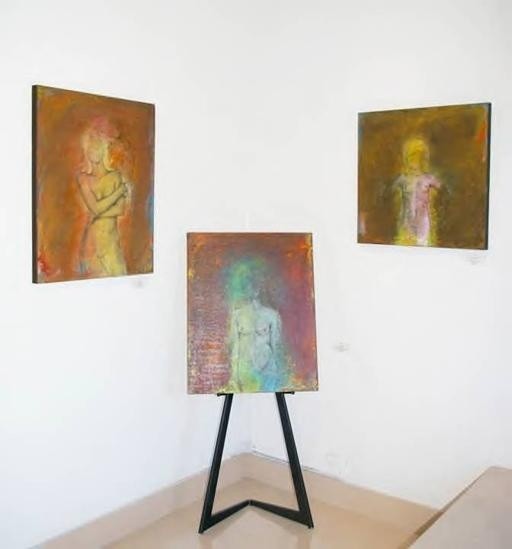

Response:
(385, 139), (441, 247)
(221, 281), (292, 392)
(76, 129), (129, 275)
